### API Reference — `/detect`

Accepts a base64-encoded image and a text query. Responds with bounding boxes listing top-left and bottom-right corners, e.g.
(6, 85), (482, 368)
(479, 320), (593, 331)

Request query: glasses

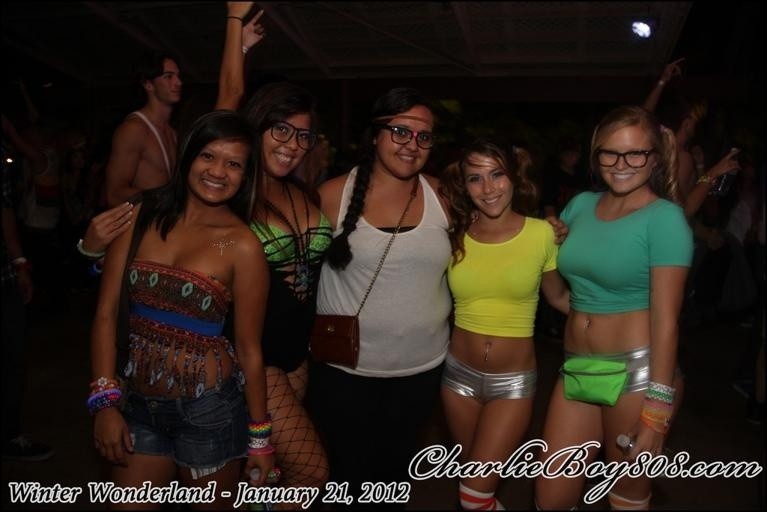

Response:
(382, 125), (438, 149)
(595, 148), (654, 168)
(271, 120), (318, 151)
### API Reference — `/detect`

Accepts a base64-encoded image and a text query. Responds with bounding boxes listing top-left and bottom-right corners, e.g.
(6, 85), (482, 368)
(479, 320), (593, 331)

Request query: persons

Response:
(0, 1), (766, 512)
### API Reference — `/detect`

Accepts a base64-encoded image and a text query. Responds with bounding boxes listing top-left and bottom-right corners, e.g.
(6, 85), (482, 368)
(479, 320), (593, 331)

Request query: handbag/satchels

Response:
(563, 357), (630, 407)
(310, 315), (359, 370)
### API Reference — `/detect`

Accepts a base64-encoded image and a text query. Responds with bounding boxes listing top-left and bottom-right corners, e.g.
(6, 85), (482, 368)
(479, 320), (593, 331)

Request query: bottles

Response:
(710, 146), (740, 198)
(614, 432), (674, 467)
(250, 467), (267, 510)
